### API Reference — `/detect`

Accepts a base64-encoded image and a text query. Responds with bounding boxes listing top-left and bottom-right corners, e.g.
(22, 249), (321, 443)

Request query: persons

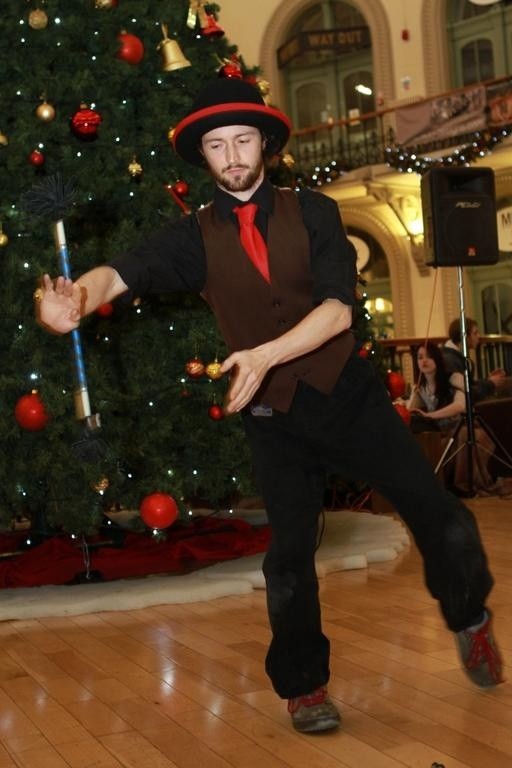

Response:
(400, 330), (474, 500)
(436, 318), (512, 413)
(34, 78), (505, 733)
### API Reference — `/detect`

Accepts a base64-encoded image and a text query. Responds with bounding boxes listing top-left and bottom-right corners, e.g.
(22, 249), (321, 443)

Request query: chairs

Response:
(440, 346), (470, 374)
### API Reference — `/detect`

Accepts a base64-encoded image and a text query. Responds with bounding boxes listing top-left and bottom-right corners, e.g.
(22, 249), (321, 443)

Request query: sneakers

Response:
(287, 685), (340, 733)
(455, 608), (501, 688)
(478, 480), (507, 496)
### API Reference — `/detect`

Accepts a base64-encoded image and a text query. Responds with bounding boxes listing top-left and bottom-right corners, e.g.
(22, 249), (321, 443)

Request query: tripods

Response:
(433, 266), (512, 498)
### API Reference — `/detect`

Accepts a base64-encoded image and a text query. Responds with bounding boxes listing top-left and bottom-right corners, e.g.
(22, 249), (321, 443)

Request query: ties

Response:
(233, 204), (271, 283)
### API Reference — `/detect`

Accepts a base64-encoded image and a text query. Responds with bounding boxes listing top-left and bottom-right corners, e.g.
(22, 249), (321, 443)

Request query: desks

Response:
(471, 394), (512, 477)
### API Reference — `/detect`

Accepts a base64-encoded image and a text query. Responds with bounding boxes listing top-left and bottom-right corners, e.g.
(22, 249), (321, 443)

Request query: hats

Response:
(172, 80), (292, 169)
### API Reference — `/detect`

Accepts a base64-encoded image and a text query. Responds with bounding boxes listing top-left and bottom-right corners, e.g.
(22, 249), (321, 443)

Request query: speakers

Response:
(419, 165), (500, 266)
